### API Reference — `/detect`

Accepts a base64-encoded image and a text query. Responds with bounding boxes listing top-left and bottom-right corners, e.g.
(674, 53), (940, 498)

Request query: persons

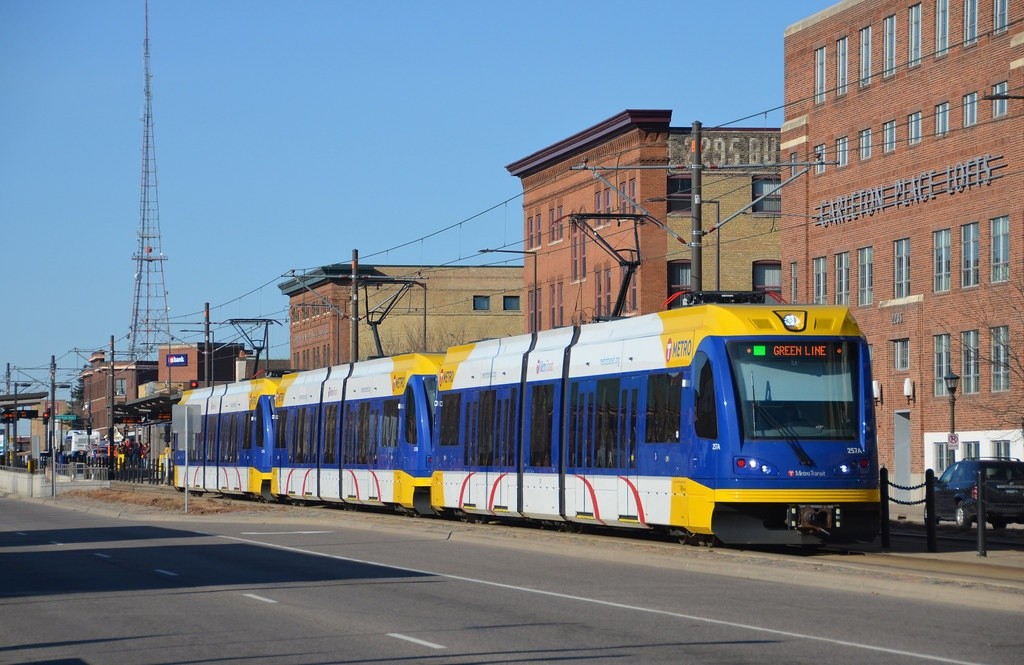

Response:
(84, 439), (152, 473)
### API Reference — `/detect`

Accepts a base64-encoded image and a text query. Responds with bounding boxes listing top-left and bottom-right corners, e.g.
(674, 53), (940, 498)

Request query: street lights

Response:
(942, 369), (960, 467)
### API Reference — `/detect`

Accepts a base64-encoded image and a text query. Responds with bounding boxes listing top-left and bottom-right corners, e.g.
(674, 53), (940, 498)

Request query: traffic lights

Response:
(42, 412), (48, 425)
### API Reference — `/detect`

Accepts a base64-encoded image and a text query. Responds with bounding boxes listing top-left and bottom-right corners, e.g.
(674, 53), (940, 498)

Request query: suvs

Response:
(923, 451), (1024, 527)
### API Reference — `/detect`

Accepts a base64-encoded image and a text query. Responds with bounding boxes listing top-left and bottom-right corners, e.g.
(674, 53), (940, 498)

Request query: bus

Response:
(427, 285), (884, 552)
(173, 347), (437, 517)
